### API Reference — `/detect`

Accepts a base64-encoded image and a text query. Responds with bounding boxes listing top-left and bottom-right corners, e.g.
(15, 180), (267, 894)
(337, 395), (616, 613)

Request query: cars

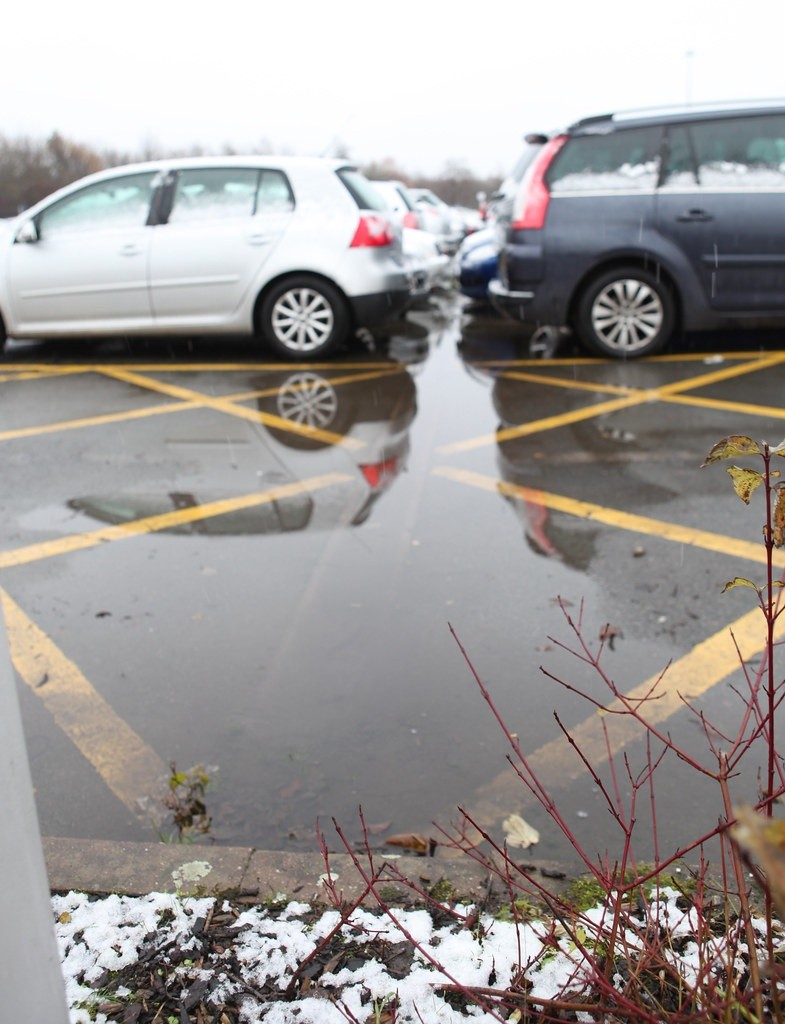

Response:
(381, 179), (510, 312)
(0, 155), (416, 361)
(50, 336), (418, 537)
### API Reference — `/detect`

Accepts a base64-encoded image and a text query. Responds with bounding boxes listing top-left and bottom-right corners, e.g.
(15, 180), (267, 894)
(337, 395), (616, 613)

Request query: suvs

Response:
(490, 101), (784, 358)
(452, 309), (784, 591)
